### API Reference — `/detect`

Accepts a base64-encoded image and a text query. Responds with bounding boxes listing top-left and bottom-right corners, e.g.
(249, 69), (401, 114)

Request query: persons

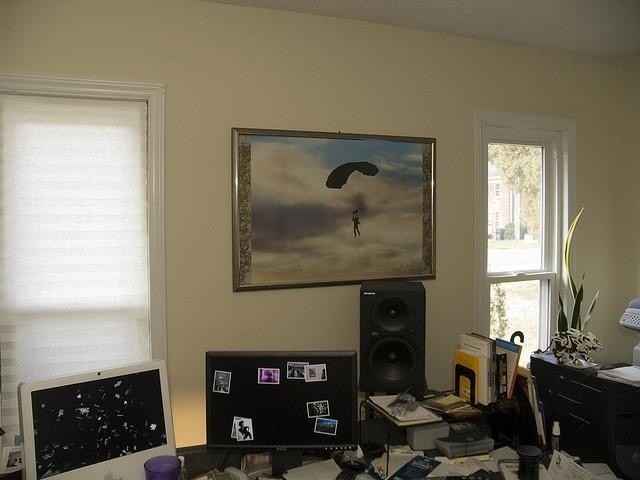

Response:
(351, 213), (362, 238)
(236, 421), (252, 441)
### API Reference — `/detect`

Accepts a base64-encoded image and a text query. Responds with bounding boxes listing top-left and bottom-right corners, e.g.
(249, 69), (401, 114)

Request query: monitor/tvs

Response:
(205, 349), (358, 480)
(17, 360), (177, 480)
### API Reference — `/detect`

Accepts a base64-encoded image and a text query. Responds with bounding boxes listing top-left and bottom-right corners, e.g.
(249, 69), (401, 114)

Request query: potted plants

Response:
(551, 202), (606, 369)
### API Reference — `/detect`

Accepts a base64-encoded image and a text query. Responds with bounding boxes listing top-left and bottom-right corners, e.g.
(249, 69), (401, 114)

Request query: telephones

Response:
(215, 466), (248, 480)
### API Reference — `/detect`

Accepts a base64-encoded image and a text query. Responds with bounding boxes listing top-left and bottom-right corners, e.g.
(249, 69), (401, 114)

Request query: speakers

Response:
(358, 280), (426, 394)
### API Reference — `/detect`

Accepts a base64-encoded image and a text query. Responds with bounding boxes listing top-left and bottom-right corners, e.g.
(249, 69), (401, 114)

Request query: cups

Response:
(144, 455), (182, 480)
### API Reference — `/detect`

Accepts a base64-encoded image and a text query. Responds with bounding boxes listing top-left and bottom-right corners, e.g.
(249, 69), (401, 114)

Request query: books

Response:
(364, 330), (523, 425)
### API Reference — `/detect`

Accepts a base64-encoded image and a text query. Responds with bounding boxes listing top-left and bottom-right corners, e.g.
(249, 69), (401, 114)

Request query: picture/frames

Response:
(229, 123), (439, 293)
